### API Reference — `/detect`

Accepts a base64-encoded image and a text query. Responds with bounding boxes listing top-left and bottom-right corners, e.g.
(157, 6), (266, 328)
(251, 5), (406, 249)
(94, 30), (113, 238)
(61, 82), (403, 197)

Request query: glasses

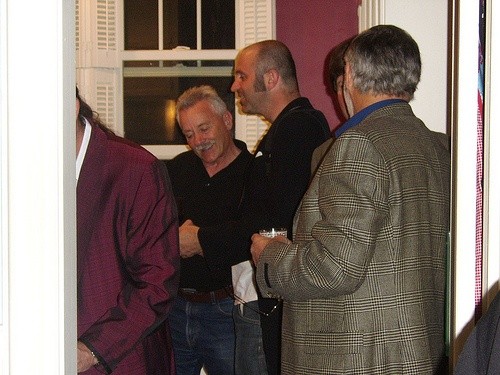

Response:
(234, 294), (282, 316)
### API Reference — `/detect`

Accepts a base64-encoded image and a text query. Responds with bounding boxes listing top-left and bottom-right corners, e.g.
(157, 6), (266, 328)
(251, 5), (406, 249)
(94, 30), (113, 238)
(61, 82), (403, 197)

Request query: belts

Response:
(178, 287), (232, 304)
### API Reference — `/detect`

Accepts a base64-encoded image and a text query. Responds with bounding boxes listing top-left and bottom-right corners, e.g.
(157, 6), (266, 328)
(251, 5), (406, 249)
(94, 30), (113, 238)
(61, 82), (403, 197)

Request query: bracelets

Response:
(92, 352), (100, 369)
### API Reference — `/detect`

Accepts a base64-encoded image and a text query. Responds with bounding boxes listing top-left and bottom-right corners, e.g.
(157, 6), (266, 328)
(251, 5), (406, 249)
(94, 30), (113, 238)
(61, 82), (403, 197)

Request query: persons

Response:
(166, 24), (450, 375)
(76, 84), (179, 375)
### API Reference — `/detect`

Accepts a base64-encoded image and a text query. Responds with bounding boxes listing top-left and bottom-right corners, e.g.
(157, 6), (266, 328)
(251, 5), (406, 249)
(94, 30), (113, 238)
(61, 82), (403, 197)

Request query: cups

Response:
(260, 227), (288, 241)
